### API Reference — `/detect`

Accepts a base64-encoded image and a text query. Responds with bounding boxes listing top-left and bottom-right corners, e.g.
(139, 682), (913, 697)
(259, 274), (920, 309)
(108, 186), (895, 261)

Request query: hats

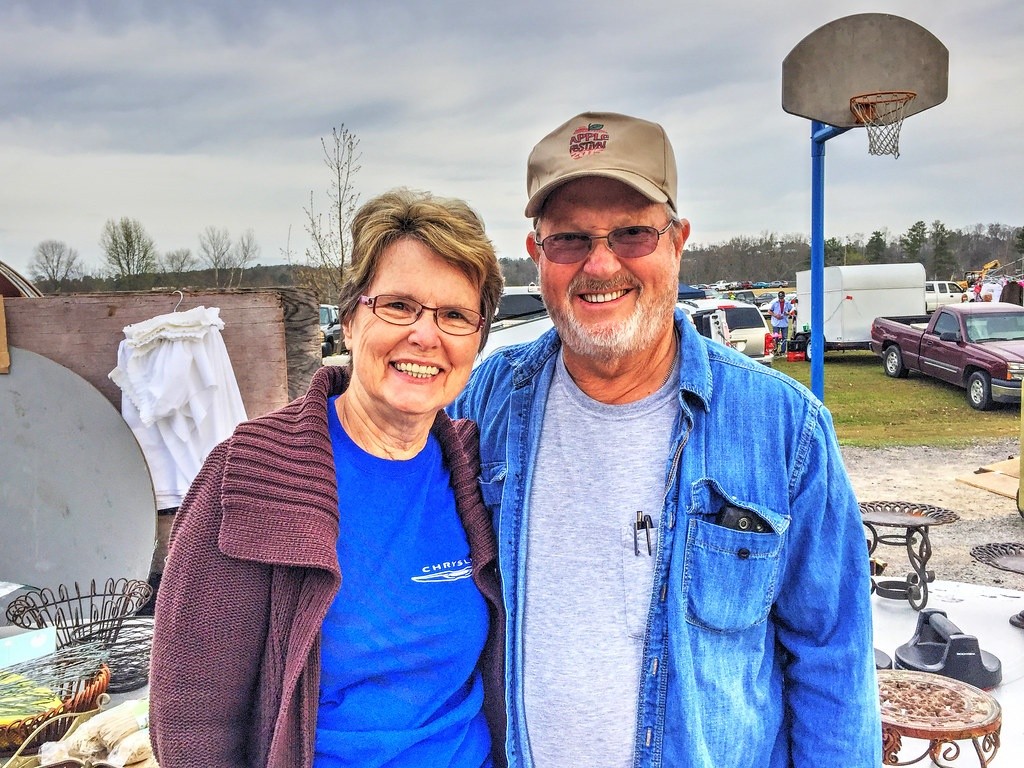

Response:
(525, 111), (677, 218)
(778, 292), (786, 298)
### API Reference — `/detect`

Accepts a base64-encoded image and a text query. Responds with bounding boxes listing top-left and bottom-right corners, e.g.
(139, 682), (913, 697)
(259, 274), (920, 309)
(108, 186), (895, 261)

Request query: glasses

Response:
(535, 219), (675, 264)
(359, 294), (486, 336)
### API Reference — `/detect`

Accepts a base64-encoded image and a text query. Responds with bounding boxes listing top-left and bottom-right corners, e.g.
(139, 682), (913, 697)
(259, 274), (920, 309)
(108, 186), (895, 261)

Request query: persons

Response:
(149, 189), (512, 768)
(727, 291), (735, 300)
(973, 276), (984, 302)
(444, 112), (886, 768)
(768, 291), (797, 356)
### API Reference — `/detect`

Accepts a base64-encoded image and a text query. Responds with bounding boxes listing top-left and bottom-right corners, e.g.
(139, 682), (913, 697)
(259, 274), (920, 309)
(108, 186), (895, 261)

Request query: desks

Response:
(0, 619), (162, 768)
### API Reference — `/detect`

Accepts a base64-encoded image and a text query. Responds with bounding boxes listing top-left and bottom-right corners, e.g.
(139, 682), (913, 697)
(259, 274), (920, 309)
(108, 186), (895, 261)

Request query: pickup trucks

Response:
(869, 302), (1024, 411)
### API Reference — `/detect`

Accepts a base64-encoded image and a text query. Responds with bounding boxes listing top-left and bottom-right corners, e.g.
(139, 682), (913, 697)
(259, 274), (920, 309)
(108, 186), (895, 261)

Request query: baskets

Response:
(0, 661), (111, 755)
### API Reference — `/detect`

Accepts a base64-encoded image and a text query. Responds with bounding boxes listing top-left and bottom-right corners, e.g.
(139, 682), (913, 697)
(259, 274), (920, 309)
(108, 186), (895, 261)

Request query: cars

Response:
(699, 288), (798, 319)
(319, 304), (350, 358)
(690, 280), (788, 292)
(471, 282), (555, 373)
(925, 281), (976, 314)
(675, 299), (774, 368)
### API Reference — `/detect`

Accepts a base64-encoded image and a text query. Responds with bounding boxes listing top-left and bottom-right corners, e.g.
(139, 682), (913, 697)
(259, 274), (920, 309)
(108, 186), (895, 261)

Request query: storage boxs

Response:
(787, 340), (806, 362)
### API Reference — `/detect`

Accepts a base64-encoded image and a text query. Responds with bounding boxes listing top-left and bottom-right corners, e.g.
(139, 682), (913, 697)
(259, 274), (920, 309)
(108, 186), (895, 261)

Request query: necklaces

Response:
(656, 355), (676, 389)
